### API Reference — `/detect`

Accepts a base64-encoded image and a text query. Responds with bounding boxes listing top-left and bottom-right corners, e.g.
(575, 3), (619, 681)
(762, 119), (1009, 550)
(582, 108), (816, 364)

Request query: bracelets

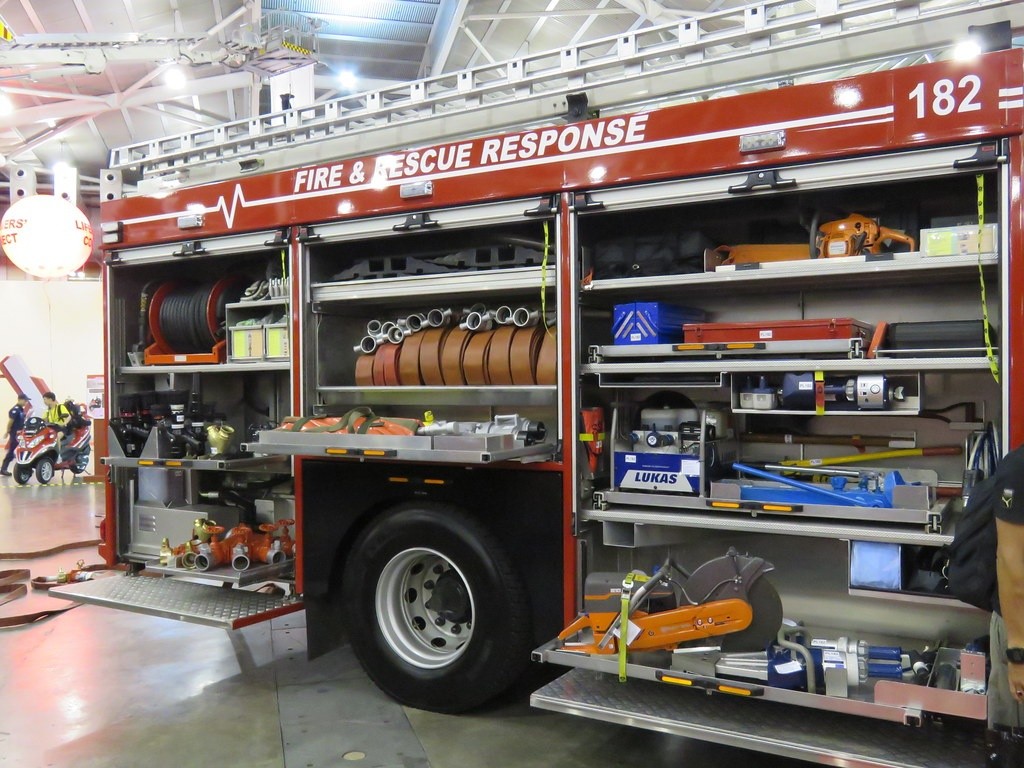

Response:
(1017, 691), (1022, 697)
(1006, 648), (1024, 665)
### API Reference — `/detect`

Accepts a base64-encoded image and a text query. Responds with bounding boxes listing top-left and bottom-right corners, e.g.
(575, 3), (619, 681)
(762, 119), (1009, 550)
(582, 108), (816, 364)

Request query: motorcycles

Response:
(13, 402), (93, 487)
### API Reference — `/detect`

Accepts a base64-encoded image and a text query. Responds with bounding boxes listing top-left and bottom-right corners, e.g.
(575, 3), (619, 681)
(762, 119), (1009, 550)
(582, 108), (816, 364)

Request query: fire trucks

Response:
(48, 1), (1024, 767)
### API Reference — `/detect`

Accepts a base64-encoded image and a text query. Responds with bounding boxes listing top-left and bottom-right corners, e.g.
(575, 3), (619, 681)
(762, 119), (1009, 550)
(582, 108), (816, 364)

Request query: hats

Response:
(19, 394), (31, 400)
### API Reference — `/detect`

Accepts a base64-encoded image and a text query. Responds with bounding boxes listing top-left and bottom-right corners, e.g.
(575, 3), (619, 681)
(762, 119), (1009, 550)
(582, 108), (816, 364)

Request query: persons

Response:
(41, 392), (71, 464)
(0, 394), (31, 476)
(987, 444), (1024, 768)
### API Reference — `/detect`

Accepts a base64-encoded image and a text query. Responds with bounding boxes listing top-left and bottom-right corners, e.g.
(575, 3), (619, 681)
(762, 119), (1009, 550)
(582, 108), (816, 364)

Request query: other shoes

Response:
(56, 455), (63, 464)
(0, 469), (12, 476)
